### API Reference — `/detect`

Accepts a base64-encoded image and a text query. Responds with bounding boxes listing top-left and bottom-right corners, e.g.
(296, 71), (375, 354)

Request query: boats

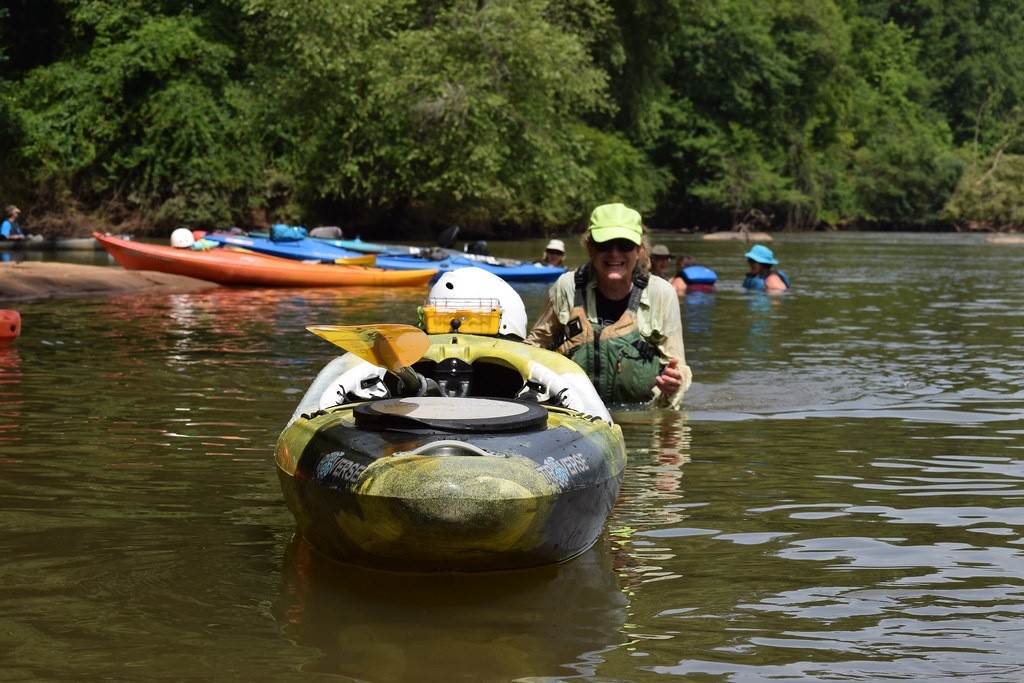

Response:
(0, 230), (135, 250)
(91, 220), (569, 289)
(274, 330), (628, 577)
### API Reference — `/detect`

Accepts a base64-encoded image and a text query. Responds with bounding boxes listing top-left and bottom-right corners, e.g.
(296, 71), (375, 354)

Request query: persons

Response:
(648, 244), (717, 300)
(743, 244), (791, 293)
(533, 238), (569, 269)
(522, 203), (692, 412)
(0, 205), (27, 240)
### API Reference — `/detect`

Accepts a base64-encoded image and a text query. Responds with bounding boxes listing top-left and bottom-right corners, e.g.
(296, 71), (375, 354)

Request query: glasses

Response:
(591, 240), (636, 252)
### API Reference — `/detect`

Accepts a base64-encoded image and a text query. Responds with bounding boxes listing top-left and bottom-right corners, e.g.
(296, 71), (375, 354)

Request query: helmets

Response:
(423, 266), (528, 340)
(170, 228), (195, 247)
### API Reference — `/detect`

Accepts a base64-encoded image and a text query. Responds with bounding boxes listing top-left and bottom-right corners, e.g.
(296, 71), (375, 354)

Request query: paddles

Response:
(198, 238), (377, 268)
(303, 323), (441, 397)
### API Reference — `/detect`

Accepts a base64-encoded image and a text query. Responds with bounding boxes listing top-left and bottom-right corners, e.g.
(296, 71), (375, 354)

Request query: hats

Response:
(545, 240), (564, 252)
(652, 244), (672, 256)
(744, 245), (777, 265)
(5, 204), (17, 214)
(587, 202), (642, 243)
